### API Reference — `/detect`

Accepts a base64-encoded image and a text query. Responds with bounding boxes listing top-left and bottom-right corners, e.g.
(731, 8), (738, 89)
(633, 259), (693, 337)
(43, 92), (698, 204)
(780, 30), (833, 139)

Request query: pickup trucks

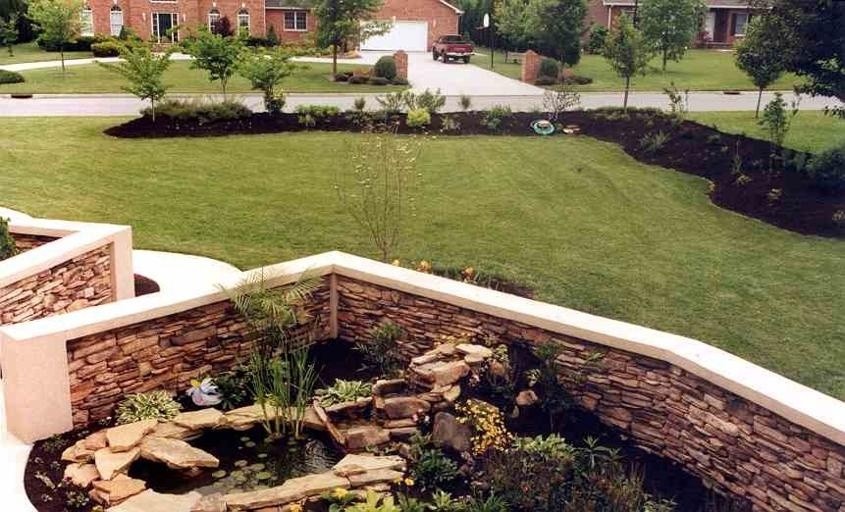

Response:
(431, 33), (474, 65)
